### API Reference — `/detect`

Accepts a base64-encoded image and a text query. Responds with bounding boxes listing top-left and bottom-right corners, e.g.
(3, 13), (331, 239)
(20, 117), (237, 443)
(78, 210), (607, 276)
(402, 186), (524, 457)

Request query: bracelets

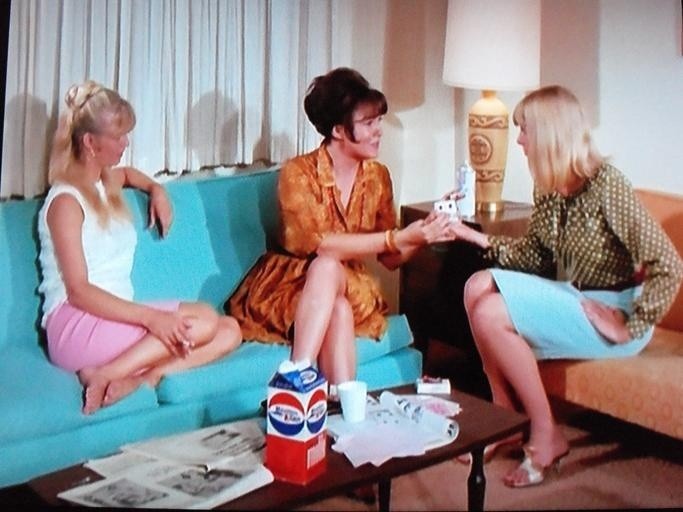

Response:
(386, 228), (404, 255)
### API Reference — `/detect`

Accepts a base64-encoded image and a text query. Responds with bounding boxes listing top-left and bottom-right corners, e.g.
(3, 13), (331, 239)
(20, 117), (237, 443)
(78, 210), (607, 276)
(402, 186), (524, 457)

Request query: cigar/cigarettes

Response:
(181, 340), (191, 346)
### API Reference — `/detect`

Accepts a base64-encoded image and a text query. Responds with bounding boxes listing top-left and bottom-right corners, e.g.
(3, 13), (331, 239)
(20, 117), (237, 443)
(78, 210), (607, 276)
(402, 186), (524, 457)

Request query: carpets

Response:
(273, 381), (683, 511)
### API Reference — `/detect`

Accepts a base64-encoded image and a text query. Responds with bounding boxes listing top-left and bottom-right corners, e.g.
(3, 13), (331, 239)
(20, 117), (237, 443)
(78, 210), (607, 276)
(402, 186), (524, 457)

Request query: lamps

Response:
(442, 2), (542, 216)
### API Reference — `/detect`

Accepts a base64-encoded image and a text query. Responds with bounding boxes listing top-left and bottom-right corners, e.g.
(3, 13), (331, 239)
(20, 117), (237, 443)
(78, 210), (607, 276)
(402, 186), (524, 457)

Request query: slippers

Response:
(456, 433), (522, 464)
(504, 445), (570, 488)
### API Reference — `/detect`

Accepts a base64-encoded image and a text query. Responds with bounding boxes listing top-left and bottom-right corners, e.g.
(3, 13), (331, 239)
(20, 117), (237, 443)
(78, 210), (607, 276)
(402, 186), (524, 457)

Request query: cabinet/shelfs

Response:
(401, 186), (544, 388)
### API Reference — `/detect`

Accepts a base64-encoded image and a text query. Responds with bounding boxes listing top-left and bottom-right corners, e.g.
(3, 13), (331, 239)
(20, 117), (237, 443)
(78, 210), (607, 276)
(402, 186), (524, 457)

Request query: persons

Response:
(29, 80), (243, 411)
(443, 86), (682, 487)
(223, 67), (458, 409)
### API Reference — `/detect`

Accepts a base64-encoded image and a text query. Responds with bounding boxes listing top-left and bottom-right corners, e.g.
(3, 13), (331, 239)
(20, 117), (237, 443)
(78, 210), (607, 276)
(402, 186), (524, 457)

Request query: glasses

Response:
(338, 113), (383, 127)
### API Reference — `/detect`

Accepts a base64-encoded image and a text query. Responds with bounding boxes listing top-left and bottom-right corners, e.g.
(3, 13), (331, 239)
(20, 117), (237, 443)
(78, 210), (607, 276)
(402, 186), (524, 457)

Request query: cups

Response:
(339, 382), (368, 423)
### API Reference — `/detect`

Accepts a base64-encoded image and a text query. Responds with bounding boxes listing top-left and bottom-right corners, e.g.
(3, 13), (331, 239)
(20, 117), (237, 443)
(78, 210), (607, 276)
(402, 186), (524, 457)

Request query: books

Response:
(318, 392), (459, 465)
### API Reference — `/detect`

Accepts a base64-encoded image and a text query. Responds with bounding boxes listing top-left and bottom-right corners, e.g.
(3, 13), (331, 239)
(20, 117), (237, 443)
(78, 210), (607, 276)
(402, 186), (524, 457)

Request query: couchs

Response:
(517, 187), (682, 439)
(0, 166), (424, 486)
